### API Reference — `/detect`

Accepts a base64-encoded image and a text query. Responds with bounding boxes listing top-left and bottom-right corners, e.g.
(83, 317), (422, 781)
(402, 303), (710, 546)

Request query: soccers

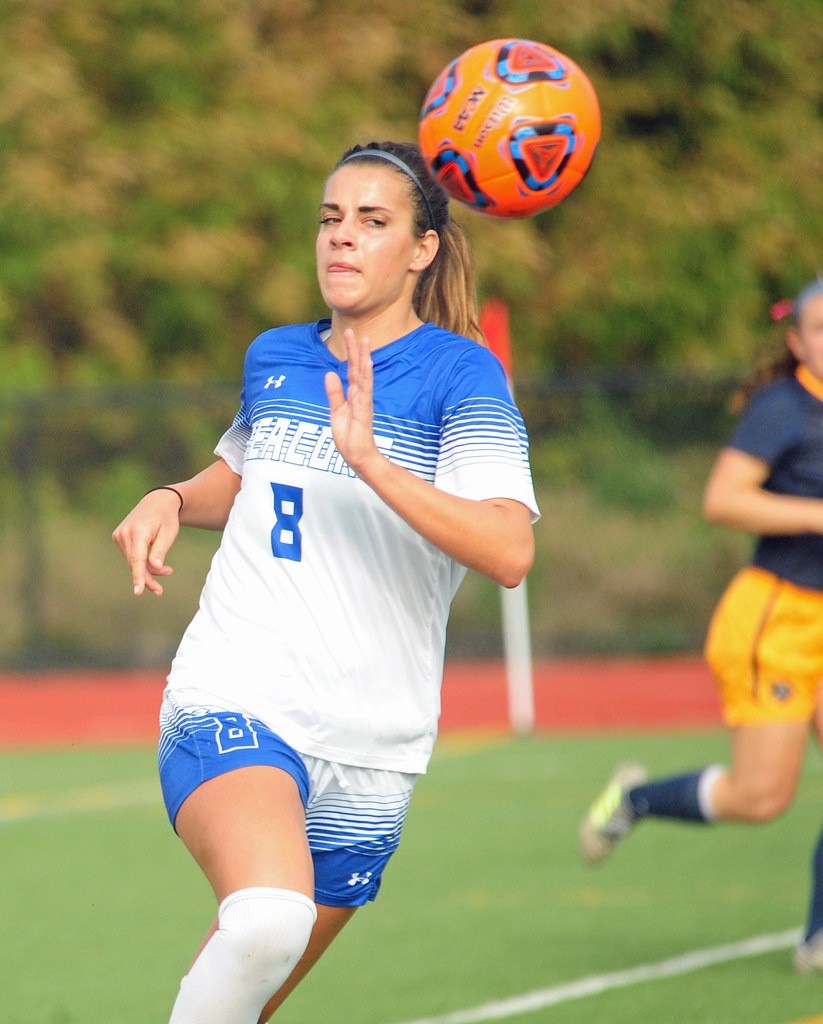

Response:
(417, 39), (603, 222)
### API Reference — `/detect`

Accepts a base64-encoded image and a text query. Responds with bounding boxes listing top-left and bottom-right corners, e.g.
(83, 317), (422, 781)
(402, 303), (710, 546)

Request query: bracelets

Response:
(141, 487), (185, 512)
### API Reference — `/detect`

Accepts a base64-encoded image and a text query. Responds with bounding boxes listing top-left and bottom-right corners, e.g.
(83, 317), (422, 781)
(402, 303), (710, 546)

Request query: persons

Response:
(107, 141), (543, 1024)
(576, 274), (823, 974)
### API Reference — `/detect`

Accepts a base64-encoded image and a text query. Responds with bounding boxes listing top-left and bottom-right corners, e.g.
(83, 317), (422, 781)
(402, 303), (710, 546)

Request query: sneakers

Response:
(796, 927), (823, 975)
(584, 766), (648, 863)
